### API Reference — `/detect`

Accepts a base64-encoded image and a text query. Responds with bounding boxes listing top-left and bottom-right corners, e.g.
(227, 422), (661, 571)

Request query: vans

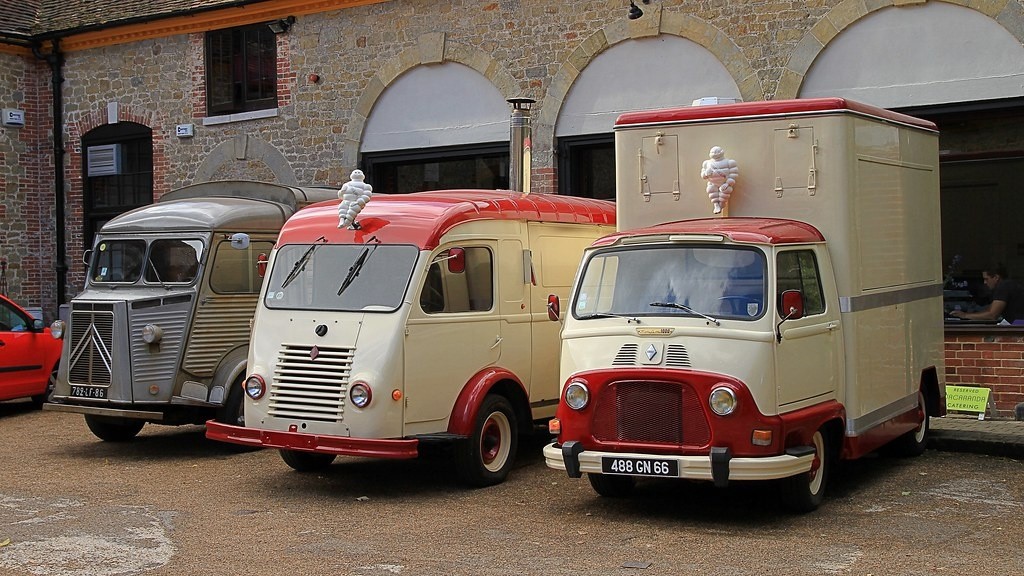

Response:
(204, 188), (620, 485)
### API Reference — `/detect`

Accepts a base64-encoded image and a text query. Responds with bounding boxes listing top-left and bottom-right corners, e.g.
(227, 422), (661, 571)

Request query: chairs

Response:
(634, 262), (675, 310)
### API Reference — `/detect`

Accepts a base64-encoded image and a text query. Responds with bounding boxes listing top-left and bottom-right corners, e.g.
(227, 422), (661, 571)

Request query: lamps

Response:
(267, 15), (295, 34)
(628, 0), (650, 19)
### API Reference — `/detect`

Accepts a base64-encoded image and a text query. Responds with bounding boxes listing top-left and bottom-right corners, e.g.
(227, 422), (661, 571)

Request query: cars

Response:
(0, 294), (63, 405)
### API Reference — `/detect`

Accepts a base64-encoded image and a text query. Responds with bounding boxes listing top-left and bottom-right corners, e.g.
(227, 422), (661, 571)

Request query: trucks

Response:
(542, 99), (945, 514)
(43, 178), (342, 442)
